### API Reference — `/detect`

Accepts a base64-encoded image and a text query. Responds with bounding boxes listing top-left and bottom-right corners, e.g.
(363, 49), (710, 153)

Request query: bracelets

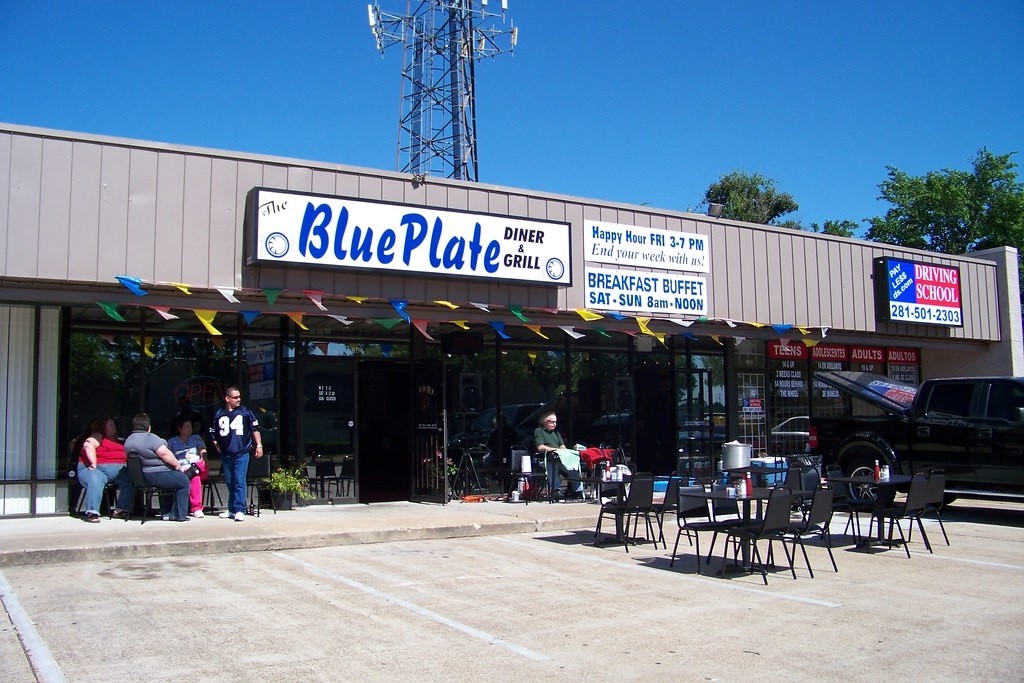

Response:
(257, 445), (263, 448)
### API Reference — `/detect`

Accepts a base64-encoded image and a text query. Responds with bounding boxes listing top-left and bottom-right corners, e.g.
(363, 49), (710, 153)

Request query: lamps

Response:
(708, 202), (724, 217)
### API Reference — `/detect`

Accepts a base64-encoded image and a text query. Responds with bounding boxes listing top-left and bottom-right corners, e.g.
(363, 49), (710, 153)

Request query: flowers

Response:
(258, 462), (317, 499)
(422, 453), (459, 475)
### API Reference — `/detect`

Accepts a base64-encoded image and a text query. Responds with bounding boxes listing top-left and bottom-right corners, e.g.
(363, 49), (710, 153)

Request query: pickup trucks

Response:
(809, 370), (1024, 512)
(449, 392), (581, 454)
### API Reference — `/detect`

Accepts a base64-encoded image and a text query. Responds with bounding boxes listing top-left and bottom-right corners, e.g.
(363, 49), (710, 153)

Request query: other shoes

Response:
(185, 517), (190, 521)
(569, 490), (580, 497)
(235, 512), (246, 521)
(554, 490), (562, 498)
(193, 510), (204, 518)
(219, 511), (234, 518)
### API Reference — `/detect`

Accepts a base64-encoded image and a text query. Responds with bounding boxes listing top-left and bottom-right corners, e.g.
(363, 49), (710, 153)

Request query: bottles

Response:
(602, 461), (623, 481)
(875, 460), (889, 481)
(735, 471), (752, 497)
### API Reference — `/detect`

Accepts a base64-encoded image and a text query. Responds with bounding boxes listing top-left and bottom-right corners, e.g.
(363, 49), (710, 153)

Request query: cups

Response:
(512, 491), (520, 500)
(728, 488), (736, 496)
(726, 488), (729, 495)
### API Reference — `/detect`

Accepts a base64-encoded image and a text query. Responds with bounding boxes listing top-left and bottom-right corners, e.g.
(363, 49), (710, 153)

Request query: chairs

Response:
(455, 447), (951, 585)
(70, 452), (273, 525)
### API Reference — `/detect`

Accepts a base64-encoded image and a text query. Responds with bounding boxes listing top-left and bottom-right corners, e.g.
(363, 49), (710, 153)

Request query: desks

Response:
(563, 475), (669, 543)
(680, 488), (811, 573)
(722, 465), (789, 519)
(824, 473), (921, 552)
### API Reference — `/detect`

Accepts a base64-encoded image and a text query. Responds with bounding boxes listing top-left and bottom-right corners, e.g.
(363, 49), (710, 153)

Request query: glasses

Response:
(547, 421), (556, 424)
(229, 395), (241, 399)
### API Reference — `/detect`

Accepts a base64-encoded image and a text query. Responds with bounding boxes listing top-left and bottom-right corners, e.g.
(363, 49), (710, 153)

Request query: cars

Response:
(769, 415), (810, 455)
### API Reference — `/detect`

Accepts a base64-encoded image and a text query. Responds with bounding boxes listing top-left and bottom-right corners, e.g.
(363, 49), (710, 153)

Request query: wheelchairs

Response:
(533, 449), (586, 504)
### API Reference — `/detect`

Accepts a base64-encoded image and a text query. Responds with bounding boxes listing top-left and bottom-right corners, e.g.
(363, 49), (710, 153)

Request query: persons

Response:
(482, 415), (520, 488)
(78, 417), (134, 523)
(69, 418), (96, 509)
(124, 413), (192, 521)
(534, 412), (591, 499)
(167, 416), (209, 518)
(209, 387), (263, 521)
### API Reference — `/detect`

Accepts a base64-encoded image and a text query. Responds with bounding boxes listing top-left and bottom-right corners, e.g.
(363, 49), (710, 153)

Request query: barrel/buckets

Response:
(721, 443), (752, 469)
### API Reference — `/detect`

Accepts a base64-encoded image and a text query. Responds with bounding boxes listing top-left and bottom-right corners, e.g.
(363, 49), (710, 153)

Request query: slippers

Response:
(89, 514), (100, 523)
(113, 511), (130, 518)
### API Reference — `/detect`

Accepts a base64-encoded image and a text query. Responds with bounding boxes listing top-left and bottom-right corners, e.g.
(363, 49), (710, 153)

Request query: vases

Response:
(258, 492), (294, 510)
(432, 474), (452, 501)
